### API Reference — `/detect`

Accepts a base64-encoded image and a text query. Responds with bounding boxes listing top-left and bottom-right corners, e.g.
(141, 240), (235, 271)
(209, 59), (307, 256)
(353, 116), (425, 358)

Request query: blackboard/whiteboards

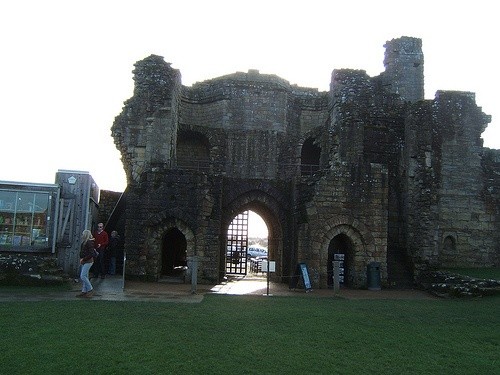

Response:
(290, 263), (311, 289)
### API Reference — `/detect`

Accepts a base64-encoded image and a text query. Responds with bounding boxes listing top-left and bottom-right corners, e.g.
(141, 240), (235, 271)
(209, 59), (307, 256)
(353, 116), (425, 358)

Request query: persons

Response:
(91, 222), (109, 280)
(76, 229), (96, 297)
(106, 230), (121, 274)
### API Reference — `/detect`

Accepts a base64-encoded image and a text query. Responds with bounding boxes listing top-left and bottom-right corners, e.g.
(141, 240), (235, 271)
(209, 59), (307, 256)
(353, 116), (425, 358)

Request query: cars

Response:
(226, 243), (268, 263)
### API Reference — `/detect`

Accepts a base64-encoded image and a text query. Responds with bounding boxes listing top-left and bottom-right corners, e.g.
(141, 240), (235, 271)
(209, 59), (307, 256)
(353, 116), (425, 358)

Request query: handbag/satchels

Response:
(93, 249), (99, 260)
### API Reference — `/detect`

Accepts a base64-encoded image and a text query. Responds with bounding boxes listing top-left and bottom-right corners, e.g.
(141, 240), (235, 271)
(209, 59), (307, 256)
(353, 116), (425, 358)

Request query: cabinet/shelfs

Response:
(0, 189), (51, 248)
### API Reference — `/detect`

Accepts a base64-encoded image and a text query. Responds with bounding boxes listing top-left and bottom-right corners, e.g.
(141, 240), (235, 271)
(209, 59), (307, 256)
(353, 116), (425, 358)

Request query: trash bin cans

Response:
(366, 264), (381, 290)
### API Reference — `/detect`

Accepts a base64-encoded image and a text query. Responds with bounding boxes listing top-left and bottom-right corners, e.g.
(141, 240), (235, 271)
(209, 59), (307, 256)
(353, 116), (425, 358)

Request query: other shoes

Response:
(76, 292), (86, 298)
(101, 276), (105, 279)
(91, 275), (98, 278)
(86, 289), (95, 297)
(107, 272), (113, 275)
